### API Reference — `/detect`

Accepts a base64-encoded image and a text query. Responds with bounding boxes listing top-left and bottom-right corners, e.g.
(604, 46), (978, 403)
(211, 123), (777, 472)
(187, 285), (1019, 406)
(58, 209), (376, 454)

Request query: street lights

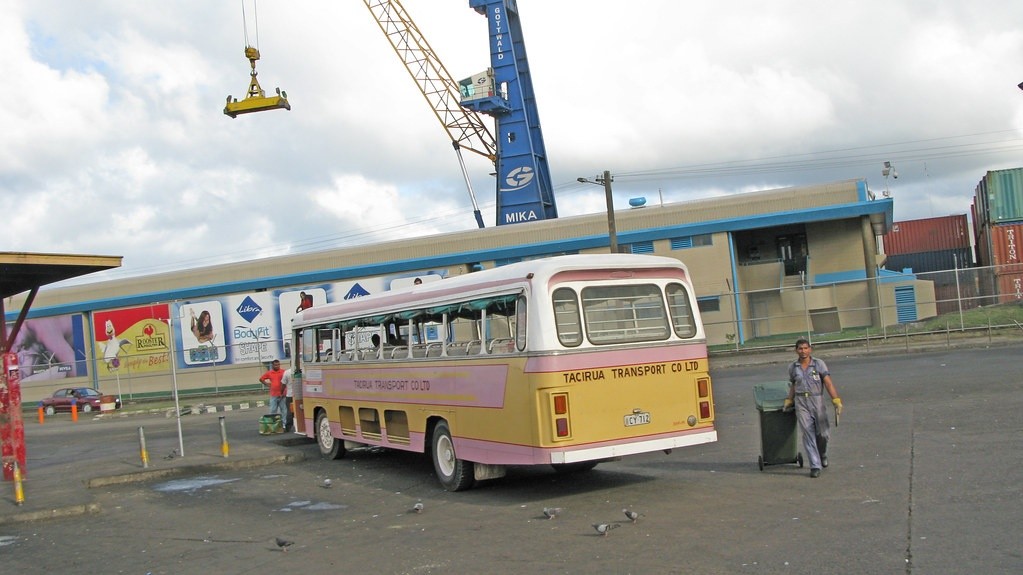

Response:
(577, 170), (618, 253)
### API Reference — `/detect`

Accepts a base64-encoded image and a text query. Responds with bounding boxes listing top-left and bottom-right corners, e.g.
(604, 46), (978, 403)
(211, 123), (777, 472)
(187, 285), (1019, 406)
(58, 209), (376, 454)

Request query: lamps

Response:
(883, 162), (898, 198)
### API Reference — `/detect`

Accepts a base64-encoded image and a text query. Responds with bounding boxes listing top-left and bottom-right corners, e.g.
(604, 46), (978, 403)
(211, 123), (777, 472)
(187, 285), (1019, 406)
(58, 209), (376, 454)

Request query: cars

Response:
(42, 386), (121, 415)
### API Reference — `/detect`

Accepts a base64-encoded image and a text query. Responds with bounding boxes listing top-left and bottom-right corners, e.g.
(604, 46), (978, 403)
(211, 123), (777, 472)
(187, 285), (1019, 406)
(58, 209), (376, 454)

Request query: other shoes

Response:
(821, 457), (828, 467)
(284, 424), (291, 433)
(810, 469), (822, 477)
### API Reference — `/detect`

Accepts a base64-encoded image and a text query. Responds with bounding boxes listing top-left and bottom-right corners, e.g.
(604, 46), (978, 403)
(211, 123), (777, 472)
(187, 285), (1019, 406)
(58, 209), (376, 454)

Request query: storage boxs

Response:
(883, 167), (1023, 314)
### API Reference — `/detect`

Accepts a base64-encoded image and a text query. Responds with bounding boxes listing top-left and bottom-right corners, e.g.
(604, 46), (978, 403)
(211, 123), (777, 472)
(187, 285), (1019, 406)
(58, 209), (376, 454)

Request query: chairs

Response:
(317, 337), (515, 360)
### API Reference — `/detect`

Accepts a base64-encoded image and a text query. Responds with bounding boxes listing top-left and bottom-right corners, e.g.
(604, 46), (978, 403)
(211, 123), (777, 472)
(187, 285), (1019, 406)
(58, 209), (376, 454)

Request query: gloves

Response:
(783, 399), (793, 413)
(832, 398), (843, 415)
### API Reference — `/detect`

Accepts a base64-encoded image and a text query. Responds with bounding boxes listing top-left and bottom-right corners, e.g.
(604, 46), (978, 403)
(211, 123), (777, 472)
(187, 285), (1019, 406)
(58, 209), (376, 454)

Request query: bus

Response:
(289, 254), (718, 492)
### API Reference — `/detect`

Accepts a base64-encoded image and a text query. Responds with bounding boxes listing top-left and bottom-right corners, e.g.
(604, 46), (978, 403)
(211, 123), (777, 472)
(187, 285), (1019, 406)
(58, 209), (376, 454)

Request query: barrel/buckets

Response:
(100, 395), (116, 414)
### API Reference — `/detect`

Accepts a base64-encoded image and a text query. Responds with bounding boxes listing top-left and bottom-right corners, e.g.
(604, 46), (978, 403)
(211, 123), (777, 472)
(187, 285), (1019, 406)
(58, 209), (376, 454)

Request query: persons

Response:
(69, 391), (77, 397)
(414, 278), (422, 285)
(280, 360), (294, 432)
(194, 311), (213, 343)
(783, 339), (843, 478)
(6, 315), (76, 379)
(372, 334), (397, 352)
(296, 292), (311, 313)
(259, 360), (287, 428)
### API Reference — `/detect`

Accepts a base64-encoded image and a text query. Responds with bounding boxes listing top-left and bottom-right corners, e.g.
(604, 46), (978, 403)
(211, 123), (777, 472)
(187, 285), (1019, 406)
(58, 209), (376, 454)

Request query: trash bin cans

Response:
(754, 379), (803, 472)
